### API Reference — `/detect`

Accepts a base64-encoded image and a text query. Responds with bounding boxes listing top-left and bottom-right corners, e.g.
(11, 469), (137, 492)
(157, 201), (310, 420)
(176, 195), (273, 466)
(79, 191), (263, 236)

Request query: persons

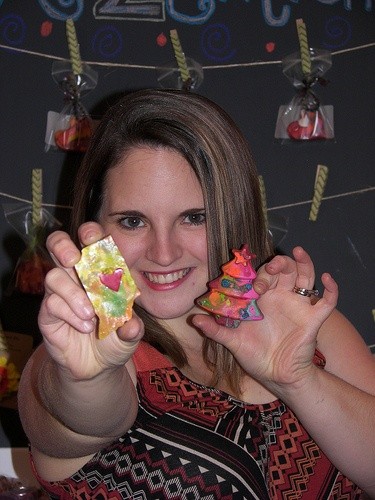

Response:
(16, 88), (375, 499)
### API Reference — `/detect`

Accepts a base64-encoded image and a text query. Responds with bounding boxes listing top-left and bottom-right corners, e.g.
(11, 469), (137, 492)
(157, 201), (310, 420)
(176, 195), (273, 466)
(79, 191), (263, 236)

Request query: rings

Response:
(295, 286), (321, 298)
